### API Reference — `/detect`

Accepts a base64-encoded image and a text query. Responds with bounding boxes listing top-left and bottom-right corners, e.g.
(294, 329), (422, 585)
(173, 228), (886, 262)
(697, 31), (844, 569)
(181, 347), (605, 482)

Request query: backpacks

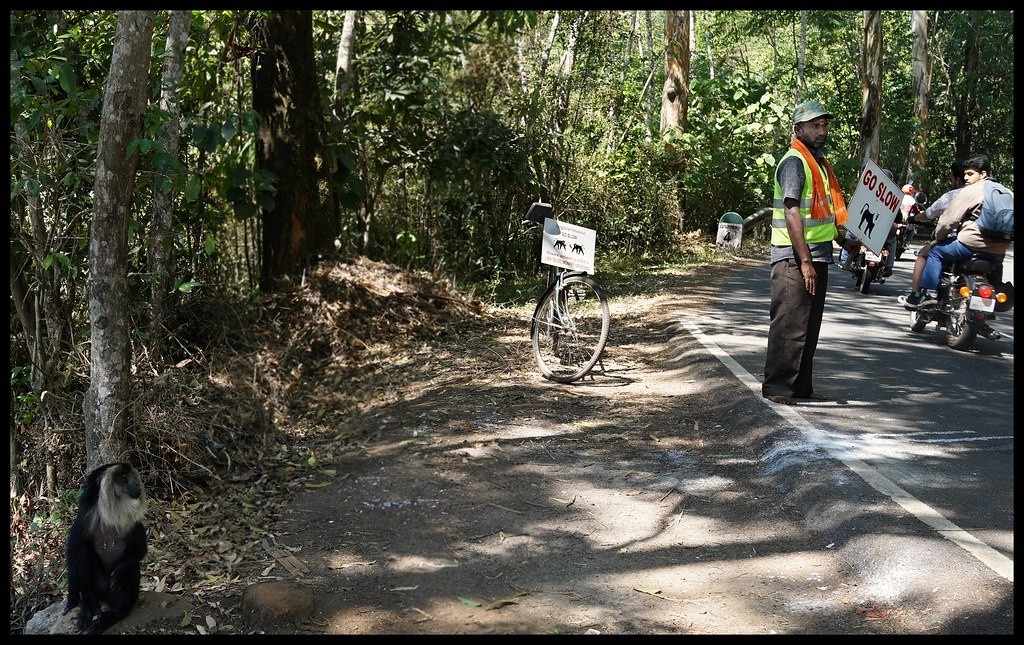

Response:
(974, 179), (1014, 242)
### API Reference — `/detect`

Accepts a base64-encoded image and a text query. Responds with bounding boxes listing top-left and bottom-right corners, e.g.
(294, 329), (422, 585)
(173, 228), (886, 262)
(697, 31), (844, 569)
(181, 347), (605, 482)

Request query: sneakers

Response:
(898, 290), (920, 309)
(917, 288), (938, 305)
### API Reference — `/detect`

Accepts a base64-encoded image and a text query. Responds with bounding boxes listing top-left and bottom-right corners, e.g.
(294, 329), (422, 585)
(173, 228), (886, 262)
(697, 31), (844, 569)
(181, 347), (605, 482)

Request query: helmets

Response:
(902, 184), (914, 195)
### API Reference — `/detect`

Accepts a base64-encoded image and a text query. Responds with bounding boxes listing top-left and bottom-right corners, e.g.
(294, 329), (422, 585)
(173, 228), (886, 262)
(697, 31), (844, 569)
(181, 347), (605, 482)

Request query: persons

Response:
(896, 179), (923, 246)
(840, 168), (897, 277)
(760, 101), (861, 406)
(918, 155), (1015, 301)
(897, 159), (965, 312)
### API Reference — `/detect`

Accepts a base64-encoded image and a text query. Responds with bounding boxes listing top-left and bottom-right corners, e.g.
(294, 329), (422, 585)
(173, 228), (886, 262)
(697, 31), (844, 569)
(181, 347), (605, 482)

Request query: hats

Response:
(792, 101), (834, 125)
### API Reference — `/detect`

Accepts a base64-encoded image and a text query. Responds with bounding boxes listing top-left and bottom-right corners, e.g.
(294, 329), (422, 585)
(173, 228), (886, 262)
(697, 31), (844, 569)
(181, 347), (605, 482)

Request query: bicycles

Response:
(524, 183), (610, 387)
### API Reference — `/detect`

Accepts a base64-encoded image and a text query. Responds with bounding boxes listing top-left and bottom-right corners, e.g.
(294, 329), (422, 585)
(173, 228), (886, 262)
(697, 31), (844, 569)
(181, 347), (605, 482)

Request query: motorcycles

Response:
(909, 191), (1002, 350)
(840, 223), (894, 295)
(890, 192), (927, 262)
